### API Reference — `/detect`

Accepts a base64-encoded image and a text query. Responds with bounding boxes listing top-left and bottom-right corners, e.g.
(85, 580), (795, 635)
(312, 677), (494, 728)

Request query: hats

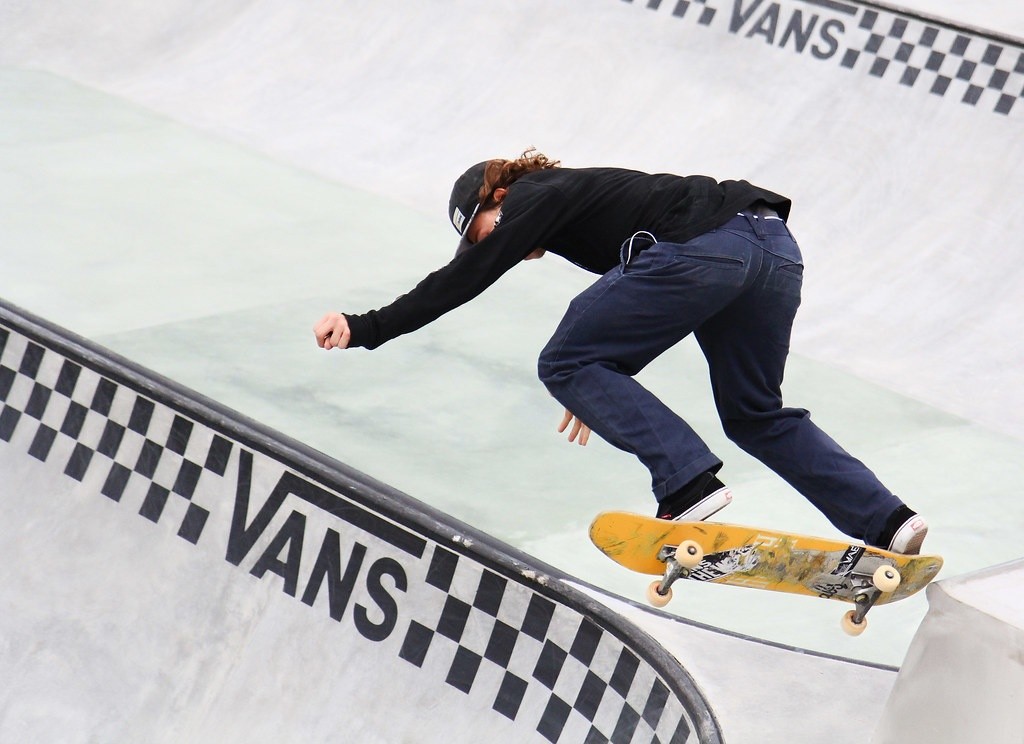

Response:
(448, 159), (506, 256)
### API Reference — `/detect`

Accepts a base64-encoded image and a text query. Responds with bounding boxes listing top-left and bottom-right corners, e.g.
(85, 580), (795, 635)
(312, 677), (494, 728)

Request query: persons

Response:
(313, 148), (928, 556)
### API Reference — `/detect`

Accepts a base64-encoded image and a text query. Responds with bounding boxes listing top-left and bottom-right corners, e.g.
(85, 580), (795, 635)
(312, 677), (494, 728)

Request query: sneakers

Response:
(874, 504), (928, 554)
(655, 469), (733, 522)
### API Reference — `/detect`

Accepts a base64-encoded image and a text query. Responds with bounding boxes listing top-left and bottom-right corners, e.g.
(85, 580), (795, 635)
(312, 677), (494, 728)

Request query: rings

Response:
(324, 331), (333, 339)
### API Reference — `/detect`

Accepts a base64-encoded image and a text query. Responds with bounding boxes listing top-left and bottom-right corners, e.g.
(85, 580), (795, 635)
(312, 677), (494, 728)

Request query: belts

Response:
(747, 204), (779, 216)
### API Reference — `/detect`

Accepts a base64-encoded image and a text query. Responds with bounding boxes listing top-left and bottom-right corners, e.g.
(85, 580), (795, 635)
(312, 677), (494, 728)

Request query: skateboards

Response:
(588, 511), (943, 635)
